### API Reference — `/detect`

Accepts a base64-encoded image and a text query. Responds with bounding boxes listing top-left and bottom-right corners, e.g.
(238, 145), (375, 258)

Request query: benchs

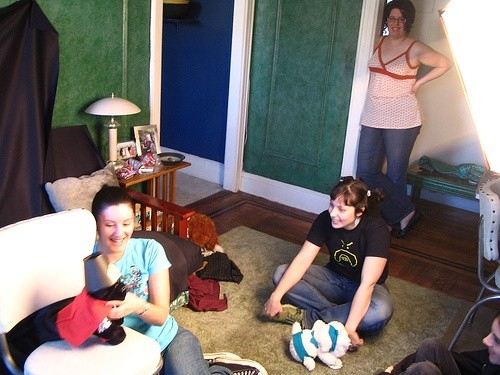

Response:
(406, 160), (479, 220)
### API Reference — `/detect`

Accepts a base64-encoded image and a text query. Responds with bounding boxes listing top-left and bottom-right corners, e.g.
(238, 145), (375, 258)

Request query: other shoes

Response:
(395, 209), (423, 238)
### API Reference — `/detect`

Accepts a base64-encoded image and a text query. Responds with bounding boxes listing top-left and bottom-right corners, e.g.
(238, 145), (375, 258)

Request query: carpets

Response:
(171, 225), (500, 375)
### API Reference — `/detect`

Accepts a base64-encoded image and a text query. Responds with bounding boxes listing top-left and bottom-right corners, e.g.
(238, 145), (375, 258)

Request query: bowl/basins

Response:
(158, 152), (186, 167)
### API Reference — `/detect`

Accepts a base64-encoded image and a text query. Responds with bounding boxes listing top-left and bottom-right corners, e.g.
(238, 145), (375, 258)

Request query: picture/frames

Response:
(117, 140), (137, 161)
(134, 125), (162, 156)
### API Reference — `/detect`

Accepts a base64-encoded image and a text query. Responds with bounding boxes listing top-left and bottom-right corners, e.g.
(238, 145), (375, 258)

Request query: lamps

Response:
(85, 93), (141, 161)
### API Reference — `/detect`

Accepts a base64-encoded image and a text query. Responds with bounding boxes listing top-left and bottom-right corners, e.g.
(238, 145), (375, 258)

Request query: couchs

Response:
(31, 125), (204, 320)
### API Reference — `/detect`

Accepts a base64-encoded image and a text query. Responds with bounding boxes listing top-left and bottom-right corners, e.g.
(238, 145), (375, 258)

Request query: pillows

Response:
(45, 162), (120, 213)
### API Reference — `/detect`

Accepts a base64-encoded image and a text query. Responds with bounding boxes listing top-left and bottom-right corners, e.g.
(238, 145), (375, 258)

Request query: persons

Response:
(92, 184), (268, 375)
(357, 0), (452, 237)
(378, 313), (500, 375)
(264, 177), (393, 351)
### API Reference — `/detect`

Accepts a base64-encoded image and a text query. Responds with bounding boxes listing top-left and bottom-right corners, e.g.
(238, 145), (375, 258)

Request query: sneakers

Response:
(208, 357), (268, 375)
(272, 304), (304, 330)
(203, 352), (241, 363)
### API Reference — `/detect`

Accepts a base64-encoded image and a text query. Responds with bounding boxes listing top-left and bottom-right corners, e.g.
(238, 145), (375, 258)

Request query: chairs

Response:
(0, 208), (163, 375)
(448, 178), (500, 352)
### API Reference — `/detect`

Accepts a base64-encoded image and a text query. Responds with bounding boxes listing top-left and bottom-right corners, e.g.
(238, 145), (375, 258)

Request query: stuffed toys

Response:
(289, 320), (351, 371)
(160, 213), (217, 251)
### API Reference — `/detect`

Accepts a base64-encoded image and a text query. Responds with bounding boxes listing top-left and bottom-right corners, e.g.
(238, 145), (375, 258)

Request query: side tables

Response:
(119, 160), (192, 229)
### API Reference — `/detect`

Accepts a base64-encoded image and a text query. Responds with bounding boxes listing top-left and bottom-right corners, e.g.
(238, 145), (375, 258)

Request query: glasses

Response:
(386, 16), (407, 23)
